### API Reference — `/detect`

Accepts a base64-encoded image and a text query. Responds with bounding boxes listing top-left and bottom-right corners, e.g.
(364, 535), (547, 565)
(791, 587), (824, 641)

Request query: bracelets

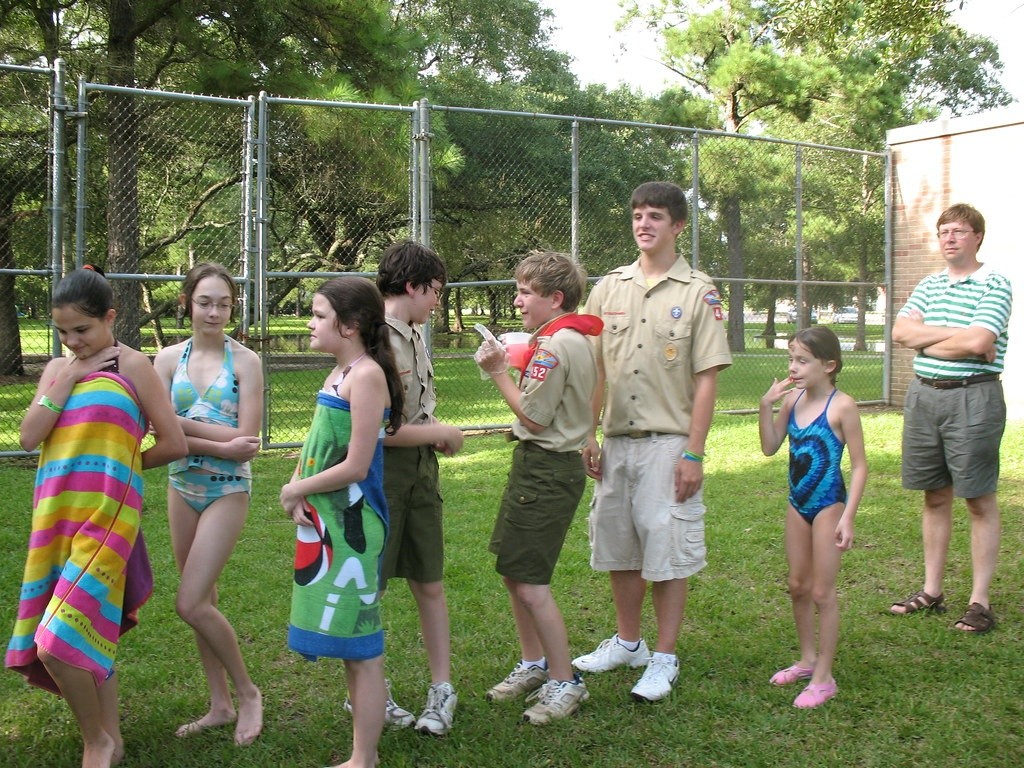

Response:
(682, 449), (703, 463)
(39, 394), (63, 414)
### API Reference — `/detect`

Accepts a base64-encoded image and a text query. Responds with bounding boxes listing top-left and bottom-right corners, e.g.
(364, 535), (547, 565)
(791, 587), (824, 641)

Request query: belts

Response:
(916, 375), (999, 388)
(615, 432), (671, 440)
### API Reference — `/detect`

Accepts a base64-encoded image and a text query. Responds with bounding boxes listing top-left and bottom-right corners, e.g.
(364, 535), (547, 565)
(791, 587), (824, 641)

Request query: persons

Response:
(280, 275), (406, 768)
(5, 262), (189, 768)
(342, 241), (464, 735)
(888, 205), (1013, 635)
(147, 260), (264, 747)
(759, 324), (868, 710)
(571, 181), (733, 701)
(474, 249), (598, 725)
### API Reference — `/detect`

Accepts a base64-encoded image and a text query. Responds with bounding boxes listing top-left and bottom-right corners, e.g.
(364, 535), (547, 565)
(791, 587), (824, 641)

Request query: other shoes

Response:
(794, 678), (838, 710)
(770, 662), (813, 686)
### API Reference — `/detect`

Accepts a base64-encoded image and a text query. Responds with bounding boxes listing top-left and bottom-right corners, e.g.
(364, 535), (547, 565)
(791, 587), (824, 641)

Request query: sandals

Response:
(953, 602), (993, 633)
(890, 590), (945, 616)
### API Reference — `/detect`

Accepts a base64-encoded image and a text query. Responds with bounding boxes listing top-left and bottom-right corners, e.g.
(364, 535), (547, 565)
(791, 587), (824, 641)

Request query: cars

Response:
(830, 306), (858, 324)
(786, 306), (818, 325)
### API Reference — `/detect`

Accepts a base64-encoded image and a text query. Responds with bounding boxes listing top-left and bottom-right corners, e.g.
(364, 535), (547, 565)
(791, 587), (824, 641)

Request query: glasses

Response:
(937, 229), (979, 239)
(191, 297), (235, 312)
(423, 281), (444, 299)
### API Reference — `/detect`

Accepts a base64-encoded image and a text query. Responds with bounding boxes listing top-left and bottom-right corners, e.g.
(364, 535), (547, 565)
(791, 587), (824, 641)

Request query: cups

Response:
(505, 332), (532, 367)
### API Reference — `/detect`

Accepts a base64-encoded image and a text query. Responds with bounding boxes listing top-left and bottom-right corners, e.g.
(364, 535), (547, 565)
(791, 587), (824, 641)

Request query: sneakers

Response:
(571, 633), (651, 672)
(415, 682), (456, 736)
(343, 689), (416, 731)
(523, 674), (590, 726)
(487, 657), (549, 702)
(630, 656), (679, 700)
